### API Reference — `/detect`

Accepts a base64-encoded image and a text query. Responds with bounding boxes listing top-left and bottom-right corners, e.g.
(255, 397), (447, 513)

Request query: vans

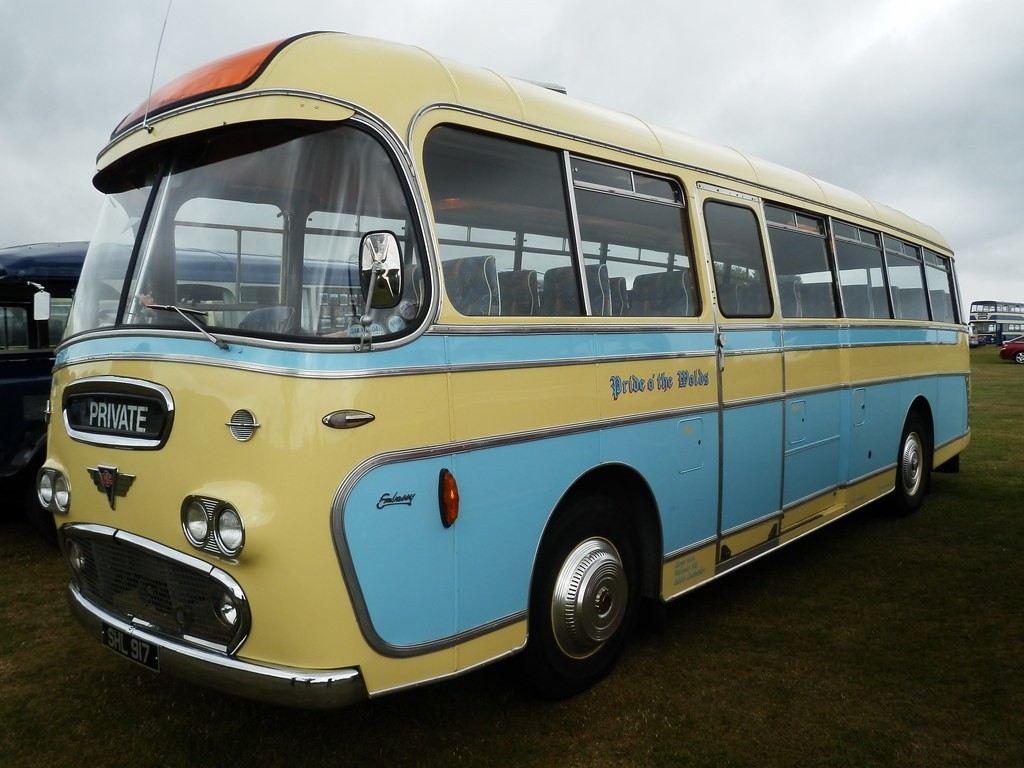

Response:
(967, 325), (979, 347)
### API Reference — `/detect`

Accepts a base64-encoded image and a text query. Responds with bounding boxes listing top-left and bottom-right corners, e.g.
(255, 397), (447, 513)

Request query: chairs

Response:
(401, 255), (948, 323)
(238, 306), (295, 335)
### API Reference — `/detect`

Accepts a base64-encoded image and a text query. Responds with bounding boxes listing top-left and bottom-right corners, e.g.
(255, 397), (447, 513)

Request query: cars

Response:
(998, 335), (1024, 365)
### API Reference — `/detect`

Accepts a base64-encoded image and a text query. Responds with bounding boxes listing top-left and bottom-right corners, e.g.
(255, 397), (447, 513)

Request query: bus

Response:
(0, 243), (367, 519)
(36, 32), (973, 709)
(968, 300), (1024, 347)
